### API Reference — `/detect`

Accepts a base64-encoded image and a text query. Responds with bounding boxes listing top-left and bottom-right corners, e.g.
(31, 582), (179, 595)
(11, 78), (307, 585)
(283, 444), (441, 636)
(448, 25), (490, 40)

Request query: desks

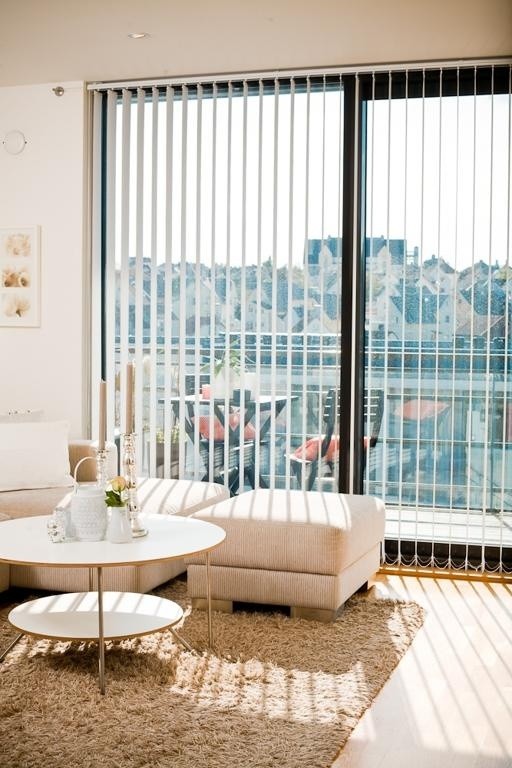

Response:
(158, 393), (299, 497)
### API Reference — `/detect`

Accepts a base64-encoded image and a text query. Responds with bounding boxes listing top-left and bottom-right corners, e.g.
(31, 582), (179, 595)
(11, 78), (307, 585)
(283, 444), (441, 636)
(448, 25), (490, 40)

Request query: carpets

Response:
(0, 580), (426, 767)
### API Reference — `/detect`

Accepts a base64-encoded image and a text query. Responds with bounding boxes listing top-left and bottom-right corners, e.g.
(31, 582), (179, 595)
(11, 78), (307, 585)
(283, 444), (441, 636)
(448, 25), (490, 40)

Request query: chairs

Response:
(284, 389), (452, 491)
(390, 403), (451, 472)
(185, 374), (213, 436)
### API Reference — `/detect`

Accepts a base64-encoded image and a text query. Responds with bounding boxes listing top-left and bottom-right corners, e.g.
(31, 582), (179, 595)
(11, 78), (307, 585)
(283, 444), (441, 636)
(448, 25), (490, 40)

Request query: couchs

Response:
(0, 411), (231, 596)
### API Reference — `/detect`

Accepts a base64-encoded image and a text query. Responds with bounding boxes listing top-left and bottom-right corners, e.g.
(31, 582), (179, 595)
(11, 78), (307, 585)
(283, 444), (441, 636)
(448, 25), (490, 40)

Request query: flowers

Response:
(105, 477), (132, 508)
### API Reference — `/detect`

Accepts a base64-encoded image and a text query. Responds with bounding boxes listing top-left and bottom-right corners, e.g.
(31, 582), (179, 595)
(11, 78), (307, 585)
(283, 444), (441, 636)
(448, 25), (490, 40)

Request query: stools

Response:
(185, 488), (387, 623)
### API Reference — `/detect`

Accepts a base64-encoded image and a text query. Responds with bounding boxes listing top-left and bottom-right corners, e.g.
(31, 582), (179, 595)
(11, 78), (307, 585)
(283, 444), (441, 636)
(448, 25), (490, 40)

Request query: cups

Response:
(234, 388), (249, 402)
(201, 383), (211, 399)
(244, 372), (258, 401)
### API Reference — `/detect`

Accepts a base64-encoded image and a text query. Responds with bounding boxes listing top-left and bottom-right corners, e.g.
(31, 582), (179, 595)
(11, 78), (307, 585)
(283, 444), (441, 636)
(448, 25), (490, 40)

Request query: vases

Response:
(106, 507), (132, 542)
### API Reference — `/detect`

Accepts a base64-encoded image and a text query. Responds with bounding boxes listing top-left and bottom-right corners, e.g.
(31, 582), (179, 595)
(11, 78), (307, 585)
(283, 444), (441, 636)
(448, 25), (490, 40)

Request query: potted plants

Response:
(200, 338), (256, 399)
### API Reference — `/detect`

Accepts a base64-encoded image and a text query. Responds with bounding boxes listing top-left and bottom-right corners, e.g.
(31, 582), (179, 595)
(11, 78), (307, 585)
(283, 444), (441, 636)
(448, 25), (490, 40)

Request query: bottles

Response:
(48, 507), (70, 544)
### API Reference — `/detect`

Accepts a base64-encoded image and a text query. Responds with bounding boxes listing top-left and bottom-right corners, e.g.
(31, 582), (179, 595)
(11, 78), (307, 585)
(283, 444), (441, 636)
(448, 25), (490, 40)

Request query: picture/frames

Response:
(0, 226), (42, 329)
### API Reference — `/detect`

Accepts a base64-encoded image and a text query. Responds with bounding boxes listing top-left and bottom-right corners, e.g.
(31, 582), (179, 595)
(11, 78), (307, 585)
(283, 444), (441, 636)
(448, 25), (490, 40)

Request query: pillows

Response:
(190, 411), (258, 441)
(1, 422), (79, 491)
(296, 435), (369, 461)
(392, 399), (450, 422)
